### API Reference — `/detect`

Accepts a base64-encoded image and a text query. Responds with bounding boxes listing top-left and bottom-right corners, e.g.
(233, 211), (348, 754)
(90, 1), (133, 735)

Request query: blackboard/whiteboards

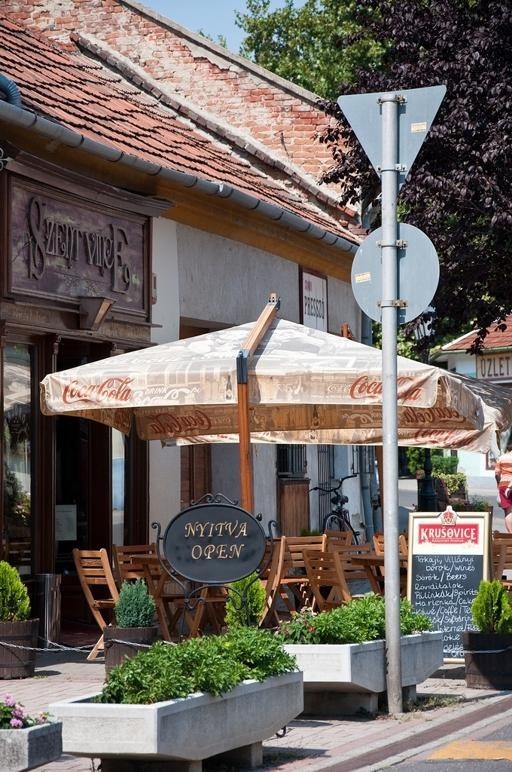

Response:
(407, 505), (491, 664)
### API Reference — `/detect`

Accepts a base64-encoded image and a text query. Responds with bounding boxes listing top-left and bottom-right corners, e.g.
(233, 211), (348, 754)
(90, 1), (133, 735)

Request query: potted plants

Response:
(462, 579), (511, 690)
(0, 560), (40, 678)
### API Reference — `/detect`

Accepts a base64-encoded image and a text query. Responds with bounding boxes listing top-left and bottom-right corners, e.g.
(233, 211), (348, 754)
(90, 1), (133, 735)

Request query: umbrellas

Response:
(161, 320), (511, 508)
(42, 293), (492, 528)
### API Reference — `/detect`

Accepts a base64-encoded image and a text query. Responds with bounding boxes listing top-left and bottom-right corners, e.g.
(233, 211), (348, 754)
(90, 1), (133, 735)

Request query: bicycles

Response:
(304, 473), (361, 561)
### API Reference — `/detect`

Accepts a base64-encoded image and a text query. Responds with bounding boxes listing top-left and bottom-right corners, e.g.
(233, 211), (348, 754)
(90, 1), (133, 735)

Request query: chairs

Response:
(490, 532), (512, 579)
(72, 530), (409, 661)
(6, 536), (32, 583)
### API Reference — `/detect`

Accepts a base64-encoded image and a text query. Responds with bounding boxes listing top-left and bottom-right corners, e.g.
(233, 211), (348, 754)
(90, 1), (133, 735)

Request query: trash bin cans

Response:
(20, 573), (62, 650)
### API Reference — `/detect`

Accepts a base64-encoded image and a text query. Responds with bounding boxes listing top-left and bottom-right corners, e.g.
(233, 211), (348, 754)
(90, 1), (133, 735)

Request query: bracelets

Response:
(506, 485), (510, 489)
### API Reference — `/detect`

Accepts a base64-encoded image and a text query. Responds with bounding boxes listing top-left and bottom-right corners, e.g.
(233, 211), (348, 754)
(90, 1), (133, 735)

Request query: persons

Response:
(494, 442), (512, 515)
(504, 480), (512, 533)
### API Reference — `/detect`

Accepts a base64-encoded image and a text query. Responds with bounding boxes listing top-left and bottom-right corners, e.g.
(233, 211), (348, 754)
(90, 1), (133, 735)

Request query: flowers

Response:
(0, 694), (47, 729)
